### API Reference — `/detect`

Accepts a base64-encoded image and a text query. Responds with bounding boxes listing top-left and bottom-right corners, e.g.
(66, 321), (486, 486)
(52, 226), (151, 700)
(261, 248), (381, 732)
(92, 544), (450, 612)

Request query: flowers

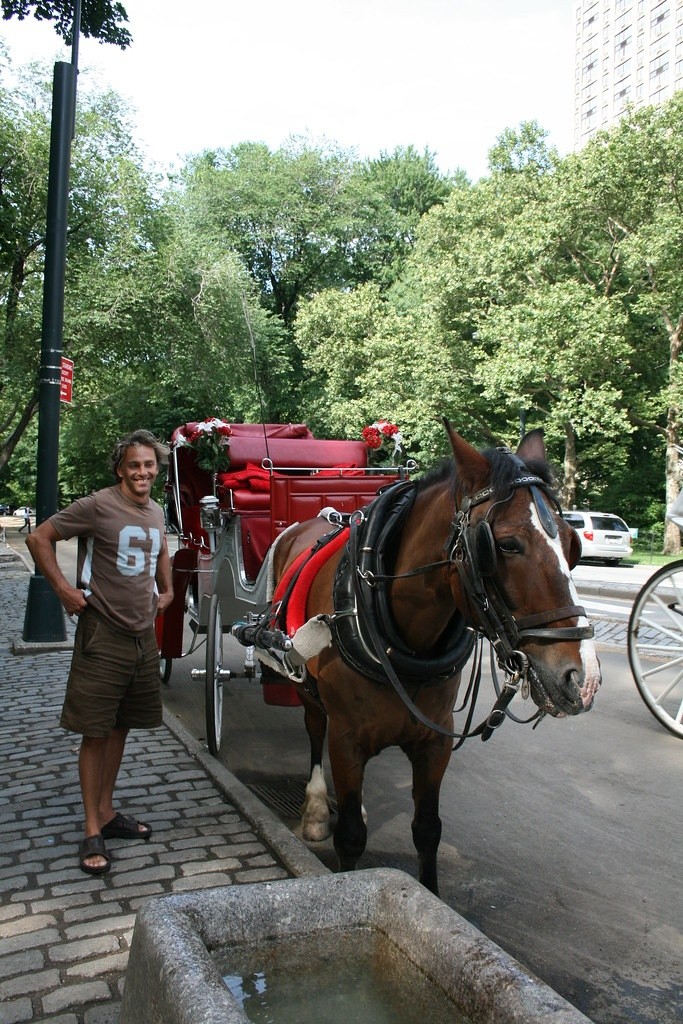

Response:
(176, 418), (234, 475)
(363, 419), (404, 474)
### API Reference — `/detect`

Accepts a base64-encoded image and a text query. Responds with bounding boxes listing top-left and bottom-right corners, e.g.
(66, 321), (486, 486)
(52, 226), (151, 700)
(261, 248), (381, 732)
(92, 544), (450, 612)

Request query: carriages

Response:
(155, 419), (602, 899)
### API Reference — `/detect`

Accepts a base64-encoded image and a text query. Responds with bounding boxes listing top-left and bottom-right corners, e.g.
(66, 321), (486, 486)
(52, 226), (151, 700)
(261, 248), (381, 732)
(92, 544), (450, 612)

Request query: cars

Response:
(12, 505), (33, 516)
(556, 508), (633, 567)
(0, 504), (13, 516)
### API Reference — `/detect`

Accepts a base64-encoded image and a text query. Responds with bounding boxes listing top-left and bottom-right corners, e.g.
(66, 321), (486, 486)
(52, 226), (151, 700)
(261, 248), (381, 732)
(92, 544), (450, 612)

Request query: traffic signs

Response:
(60, 355), (73, 405)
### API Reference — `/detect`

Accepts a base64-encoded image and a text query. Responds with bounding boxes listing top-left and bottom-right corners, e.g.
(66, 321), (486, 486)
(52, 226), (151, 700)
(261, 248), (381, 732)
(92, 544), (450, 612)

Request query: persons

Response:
(25, 431), (174, 874)
(19, 507), (32, 534)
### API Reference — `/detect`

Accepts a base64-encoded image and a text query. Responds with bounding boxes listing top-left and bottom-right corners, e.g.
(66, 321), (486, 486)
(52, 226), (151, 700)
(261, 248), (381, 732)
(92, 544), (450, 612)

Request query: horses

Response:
(271, 416), (605, 901)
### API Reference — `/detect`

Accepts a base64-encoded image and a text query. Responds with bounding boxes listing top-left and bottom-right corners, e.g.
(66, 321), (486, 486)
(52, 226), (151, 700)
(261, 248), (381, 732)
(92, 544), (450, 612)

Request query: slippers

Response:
(80, 837), (111, 874)
(97, 811), (152, 839)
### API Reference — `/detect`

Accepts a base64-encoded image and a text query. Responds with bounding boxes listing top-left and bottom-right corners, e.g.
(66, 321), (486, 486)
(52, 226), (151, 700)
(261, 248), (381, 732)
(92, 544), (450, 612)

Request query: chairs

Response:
(215, 435), (370, 513)
